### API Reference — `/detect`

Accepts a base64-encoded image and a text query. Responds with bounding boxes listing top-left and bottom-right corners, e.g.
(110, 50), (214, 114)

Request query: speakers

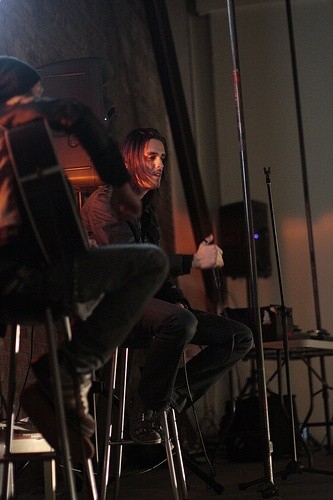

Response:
(34, 57), (117, 191)
(218, 200), (273, 280)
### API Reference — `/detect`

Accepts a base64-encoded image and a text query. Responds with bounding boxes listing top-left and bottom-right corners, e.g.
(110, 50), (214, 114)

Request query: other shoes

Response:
(131, 414), (161, 445)
(152, 422), (175, 453)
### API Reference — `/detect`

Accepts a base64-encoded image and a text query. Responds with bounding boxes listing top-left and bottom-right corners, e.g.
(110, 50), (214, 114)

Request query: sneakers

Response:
(19, 384), (95, 461)
(29, 352), (98, 438)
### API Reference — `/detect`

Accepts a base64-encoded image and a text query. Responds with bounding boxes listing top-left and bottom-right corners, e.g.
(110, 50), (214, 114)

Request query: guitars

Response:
(5, 116), (91, 267)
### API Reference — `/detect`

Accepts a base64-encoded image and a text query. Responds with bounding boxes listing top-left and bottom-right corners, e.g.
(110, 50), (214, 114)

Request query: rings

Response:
(203, 239), (209, 244)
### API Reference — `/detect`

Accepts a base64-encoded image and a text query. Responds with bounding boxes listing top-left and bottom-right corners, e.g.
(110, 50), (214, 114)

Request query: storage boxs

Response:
(221, 304), (293, 342)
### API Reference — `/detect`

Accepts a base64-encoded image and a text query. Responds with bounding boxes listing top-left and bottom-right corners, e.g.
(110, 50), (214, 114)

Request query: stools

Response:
(101, 341), (189, 499)
(0, 296), (99, 500)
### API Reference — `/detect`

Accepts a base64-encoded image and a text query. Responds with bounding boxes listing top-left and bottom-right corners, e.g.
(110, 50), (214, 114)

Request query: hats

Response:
(0, 56), (43, 104)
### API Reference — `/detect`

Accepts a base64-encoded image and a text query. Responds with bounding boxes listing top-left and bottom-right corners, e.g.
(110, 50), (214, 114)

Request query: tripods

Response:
(239, 168), (333, 490)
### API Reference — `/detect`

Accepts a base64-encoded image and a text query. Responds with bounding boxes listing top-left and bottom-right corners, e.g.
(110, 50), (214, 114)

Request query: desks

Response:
(244, 338), (333, 475)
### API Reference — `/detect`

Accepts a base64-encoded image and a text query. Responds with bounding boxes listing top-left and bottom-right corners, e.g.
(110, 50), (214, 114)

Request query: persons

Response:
(80, 128), (254, 445)
(0, 56), (168, 462)
